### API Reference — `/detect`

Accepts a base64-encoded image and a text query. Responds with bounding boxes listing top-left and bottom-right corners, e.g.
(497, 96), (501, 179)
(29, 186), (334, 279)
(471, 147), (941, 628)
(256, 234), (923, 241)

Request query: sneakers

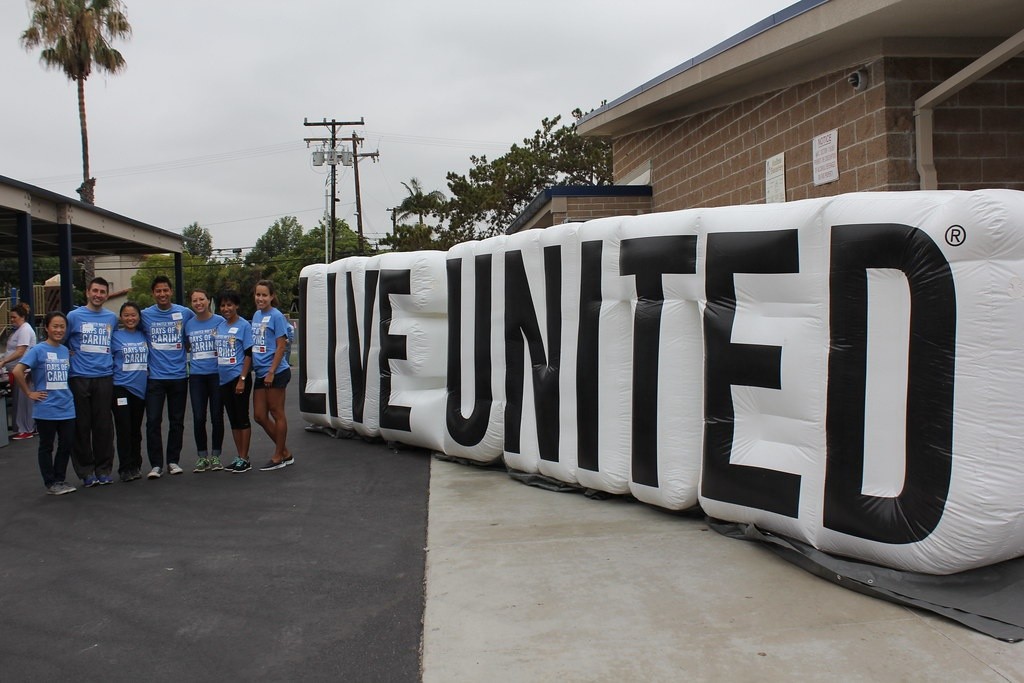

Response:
(210, 456), (224, 471)
(225, 456), (246, 471)
(120, 470), (134, 482)
(95, 473), (113, 485)
(259, 458), (286, 471)
(167, 463), (183, 475)
(147, 467), (164, 479)
(193, 457), (210, 473)
(131, 468), (143, 479)
(46, 484), (69, 495)
(59, 481), (77, 492)
(27, 432), (34, 438)
(82, 474), (99, 488)
(232, 456), (251, 473)
(284, 455), (295, 465)
(12, 432), (31, 440)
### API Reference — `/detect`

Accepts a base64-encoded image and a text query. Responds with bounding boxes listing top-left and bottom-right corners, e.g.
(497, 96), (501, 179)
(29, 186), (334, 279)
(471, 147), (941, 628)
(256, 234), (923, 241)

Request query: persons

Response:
(59, 277), (119, 488)
(213, 289), (254, 472)
(182, 289), (226, 473)
(284, 313), (294, 367)
(12, 311), (77, 496)
(251, 280), (295, 471)
(0, 302), (39, 440)
(110, 301), (150, 482)
(135, 275), (197, 478)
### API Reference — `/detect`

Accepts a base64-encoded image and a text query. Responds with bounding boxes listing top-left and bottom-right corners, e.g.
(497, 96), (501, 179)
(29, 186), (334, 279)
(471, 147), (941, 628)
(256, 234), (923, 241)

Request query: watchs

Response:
(240, 376), (245, 381)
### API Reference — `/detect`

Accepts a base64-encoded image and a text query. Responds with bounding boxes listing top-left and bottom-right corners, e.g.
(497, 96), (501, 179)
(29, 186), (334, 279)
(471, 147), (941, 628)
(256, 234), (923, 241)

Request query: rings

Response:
(240, 392), (242, 393)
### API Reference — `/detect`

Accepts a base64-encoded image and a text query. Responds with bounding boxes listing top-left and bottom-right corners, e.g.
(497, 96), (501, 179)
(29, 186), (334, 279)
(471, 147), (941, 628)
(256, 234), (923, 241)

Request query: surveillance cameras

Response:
(848, 70), (868, 91)
